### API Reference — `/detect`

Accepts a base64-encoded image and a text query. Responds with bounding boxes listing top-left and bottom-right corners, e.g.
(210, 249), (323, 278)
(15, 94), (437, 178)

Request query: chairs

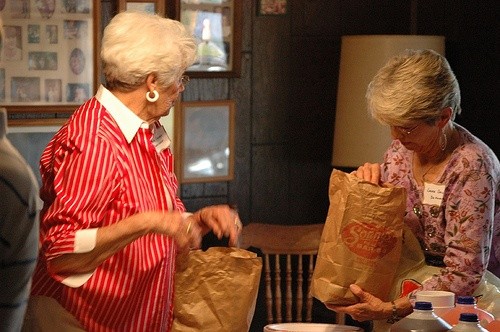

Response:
(239, 222), (345, 325)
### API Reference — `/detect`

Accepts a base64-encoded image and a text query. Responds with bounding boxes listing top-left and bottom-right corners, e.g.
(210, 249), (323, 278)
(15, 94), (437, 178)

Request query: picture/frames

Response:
(181, 100), (236, 185)
(174, 0), (242, 80)
(256, 0), (289, 17)
(116, 0), (167, 18)
(158, 100), (180, 181)
(0, 0), (101, 134)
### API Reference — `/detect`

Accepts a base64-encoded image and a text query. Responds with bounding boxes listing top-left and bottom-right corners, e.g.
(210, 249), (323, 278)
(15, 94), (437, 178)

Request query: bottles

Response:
(389, 291), (500, 332)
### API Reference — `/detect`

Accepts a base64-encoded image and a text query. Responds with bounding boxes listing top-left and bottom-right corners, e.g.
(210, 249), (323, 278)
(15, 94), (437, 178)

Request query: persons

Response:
(0, 107), (39, 332)
(323, 50), (500, 322)
(23, 10), (243, 332)
(0, 0), (86, 103)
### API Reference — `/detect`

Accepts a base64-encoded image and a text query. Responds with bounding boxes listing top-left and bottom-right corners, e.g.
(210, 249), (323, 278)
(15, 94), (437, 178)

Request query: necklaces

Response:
(421, 133), (447, 185)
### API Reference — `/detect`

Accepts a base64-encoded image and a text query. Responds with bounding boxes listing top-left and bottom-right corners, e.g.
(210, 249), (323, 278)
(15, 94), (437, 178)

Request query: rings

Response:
(186, 222), (191, 235)
(234, 218), (240, 232)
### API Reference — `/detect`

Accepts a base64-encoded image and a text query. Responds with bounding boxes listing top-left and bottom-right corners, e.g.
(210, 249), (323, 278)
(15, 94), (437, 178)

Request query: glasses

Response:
(178, 75), (191, 86)
(396, 120), (424, 138)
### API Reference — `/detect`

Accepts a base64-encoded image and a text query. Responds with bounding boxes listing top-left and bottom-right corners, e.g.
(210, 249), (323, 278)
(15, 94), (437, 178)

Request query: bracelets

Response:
(199, 208), (205, 225)
(392, 301), (396, 319)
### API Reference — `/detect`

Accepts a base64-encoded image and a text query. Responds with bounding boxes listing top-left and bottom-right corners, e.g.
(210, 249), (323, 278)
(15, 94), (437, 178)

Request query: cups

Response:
(416, 290), (455, 315)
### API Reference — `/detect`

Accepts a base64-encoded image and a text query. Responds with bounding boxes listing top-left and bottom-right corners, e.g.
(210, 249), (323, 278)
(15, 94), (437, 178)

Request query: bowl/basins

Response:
(263, 322), (365, 332)
